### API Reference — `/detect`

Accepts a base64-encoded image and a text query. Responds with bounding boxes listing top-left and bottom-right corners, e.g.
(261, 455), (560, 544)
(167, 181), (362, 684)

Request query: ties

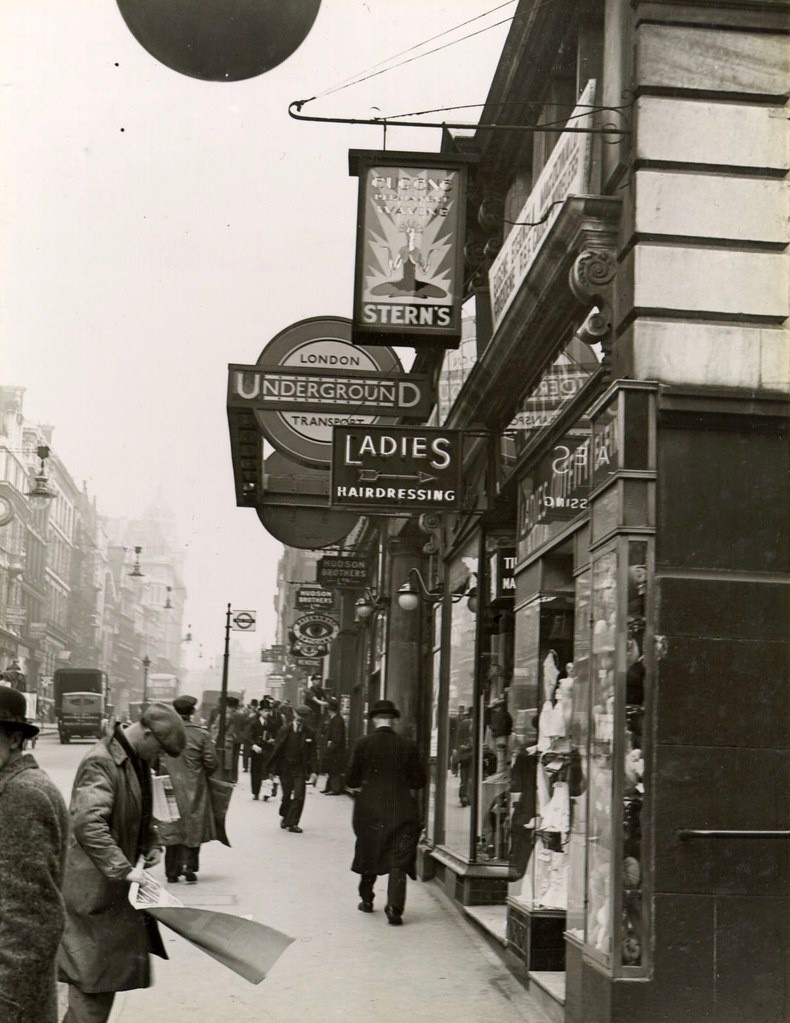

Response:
(264, 720), (266, 726)
(296, 725), (300, 732)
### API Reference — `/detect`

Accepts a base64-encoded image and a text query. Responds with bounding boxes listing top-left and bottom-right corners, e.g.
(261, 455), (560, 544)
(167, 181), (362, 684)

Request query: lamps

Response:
(396, 567), (444, 612)
(450, 587), (477, 613)
(353, 595), (390, 618)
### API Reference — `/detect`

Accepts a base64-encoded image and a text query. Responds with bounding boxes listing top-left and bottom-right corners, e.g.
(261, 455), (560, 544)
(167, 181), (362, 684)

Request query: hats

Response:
(143, 703), (187, 758)
(465, 707), (473, 716)
(0, 685), (39, 739)
(311, 672), (322, 681)
(258, 700), (272, 710)
(173, 695), (197, 709)
(369, 700), (400, 718)
(295, 704), (312, 716)
(326, 700), (338, 711)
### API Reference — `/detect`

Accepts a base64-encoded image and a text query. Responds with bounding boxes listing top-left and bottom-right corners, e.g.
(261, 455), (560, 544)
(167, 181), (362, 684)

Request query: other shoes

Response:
(167, 876), (180, 883)
(320, 790), (339, 795)
(281, 817), (290, 828)
(461, 797), (472, 807)
(186, 873), (197, 884)
(358, 901), (373, 913)
(384, 906), (402, 924)
(289, 825), (302, 833)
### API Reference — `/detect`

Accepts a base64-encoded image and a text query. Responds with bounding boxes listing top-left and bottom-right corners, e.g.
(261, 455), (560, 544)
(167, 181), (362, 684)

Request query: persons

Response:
(210, 672), (345, 833)
(507, 714), (540, 883)
(448, 704), (497, 808)
(152, 695), (220, 883)
(54, 701), (186, 1023)
(346, 700), (427, 926)
(0, 685), (69, 1023)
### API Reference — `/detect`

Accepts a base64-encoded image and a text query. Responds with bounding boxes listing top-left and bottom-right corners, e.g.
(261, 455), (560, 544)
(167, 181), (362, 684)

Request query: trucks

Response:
(51, 667), (111, 744)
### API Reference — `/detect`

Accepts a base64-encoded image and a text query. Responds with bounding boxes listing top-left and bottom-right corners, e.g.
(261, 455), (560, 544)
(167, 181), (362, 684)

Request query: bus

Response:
(147, 673), (178, 704)
